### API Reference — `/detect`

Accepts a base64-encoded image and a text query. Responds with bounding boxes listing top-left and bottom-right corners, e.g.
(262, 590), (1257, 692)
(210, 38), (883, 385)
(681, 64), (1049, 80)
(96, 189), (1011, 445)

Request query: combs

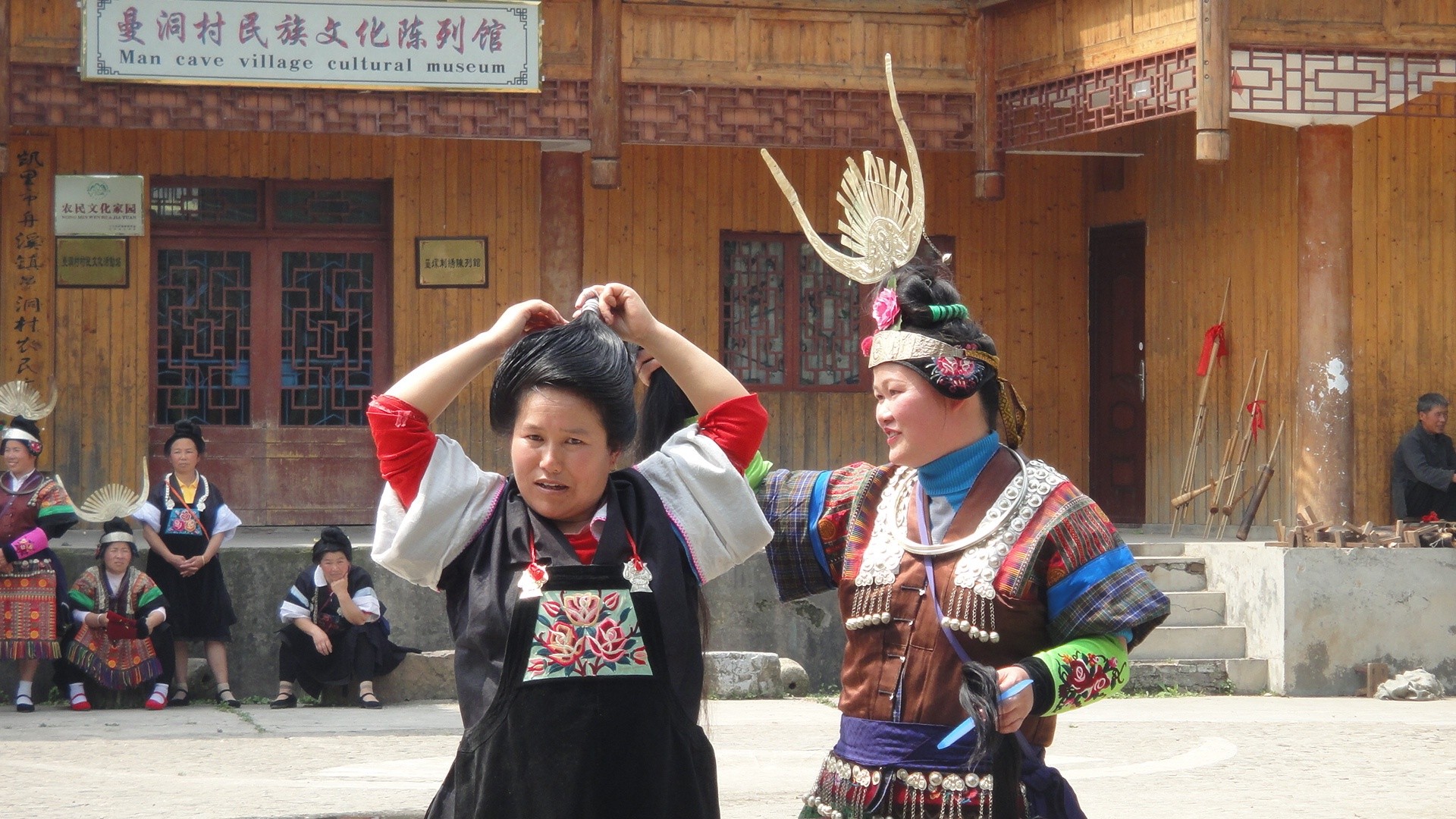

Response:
(936, 677), (1036, 752)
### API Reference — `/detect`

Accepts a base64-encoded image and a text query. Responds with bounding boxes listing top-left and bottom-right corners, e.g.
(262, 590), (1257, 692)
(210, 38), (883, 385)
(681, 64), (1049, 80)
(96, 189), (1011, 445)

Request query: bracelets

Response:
(97, 613), (105, 628)
(201, 554), (206, 567)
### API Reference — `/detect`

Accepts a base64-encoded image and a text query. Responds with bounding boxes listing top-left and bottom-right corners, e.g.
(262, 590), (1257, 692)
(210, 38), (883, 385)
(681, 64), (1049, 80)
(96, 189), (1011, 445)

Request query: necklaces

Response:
(886, 441), (1030, 562)
(0, 468), (45, 495)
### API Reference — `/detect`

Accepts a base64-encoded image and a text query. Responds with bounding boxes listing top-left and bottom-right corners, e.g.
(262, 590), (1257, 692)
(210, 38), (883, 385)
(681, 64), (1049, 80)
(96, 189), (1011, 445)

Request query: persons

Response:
(633, 265), (1175, 819)
(268, 526), (423, 711)
(1390, 392), (1456, 523)
(364, 280), (776, 819)
(0, 414), (79, 714)
(131, 418), (244, 709)
(54, 515), (177, 711)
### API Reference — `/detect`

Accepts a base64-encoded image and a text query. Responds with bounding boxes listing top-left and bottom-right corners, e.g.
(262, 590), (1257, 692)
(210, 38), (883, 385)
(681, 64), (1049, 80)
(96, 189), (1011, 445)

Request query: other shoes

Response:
(69, 692), (92, 711)
(16, 694), (35, 712)
(356, 693), (382, 709)
(168, 689), (189, 706)
(217, 689), (241, 708)
(270, 692), (297, 709)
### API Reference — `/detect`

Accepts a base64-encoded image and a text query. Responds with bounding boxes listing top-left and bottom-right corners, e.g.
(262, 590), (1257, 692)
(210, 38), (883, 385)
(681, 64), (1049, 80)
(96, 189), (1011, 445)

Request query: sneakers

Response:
(145, 691), (169, 710)
